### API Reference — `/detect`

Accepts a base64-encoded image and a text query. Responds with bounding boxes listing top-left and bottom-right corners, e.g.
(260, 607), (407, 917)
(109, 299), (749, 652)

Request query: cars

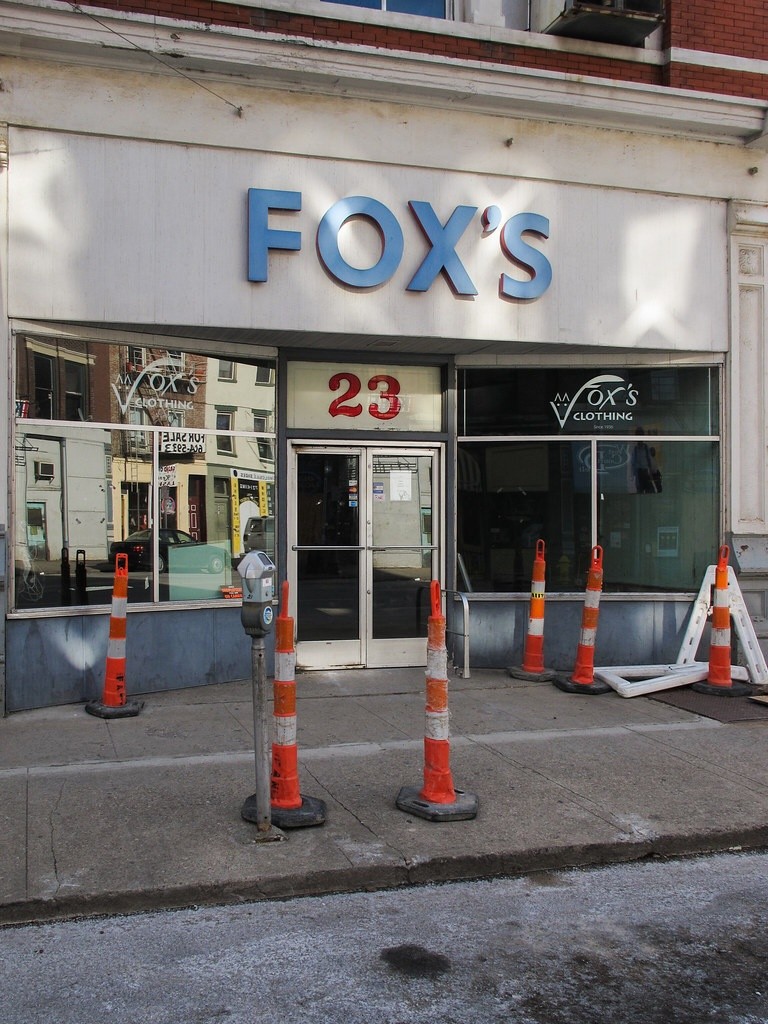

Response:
(108, 528), (229, 576)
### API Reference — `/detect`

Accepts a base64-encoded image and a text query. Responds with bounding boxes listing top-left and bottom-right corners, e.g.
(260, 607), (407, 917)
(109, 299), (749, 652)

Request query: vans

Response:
(238, 514), (275, 566)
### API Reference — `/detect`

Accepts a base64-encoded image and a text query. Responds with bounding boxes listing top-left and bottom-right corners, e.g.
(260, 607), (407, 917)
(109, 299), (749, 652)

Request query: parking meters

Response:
(236, 550), (292, 844)
